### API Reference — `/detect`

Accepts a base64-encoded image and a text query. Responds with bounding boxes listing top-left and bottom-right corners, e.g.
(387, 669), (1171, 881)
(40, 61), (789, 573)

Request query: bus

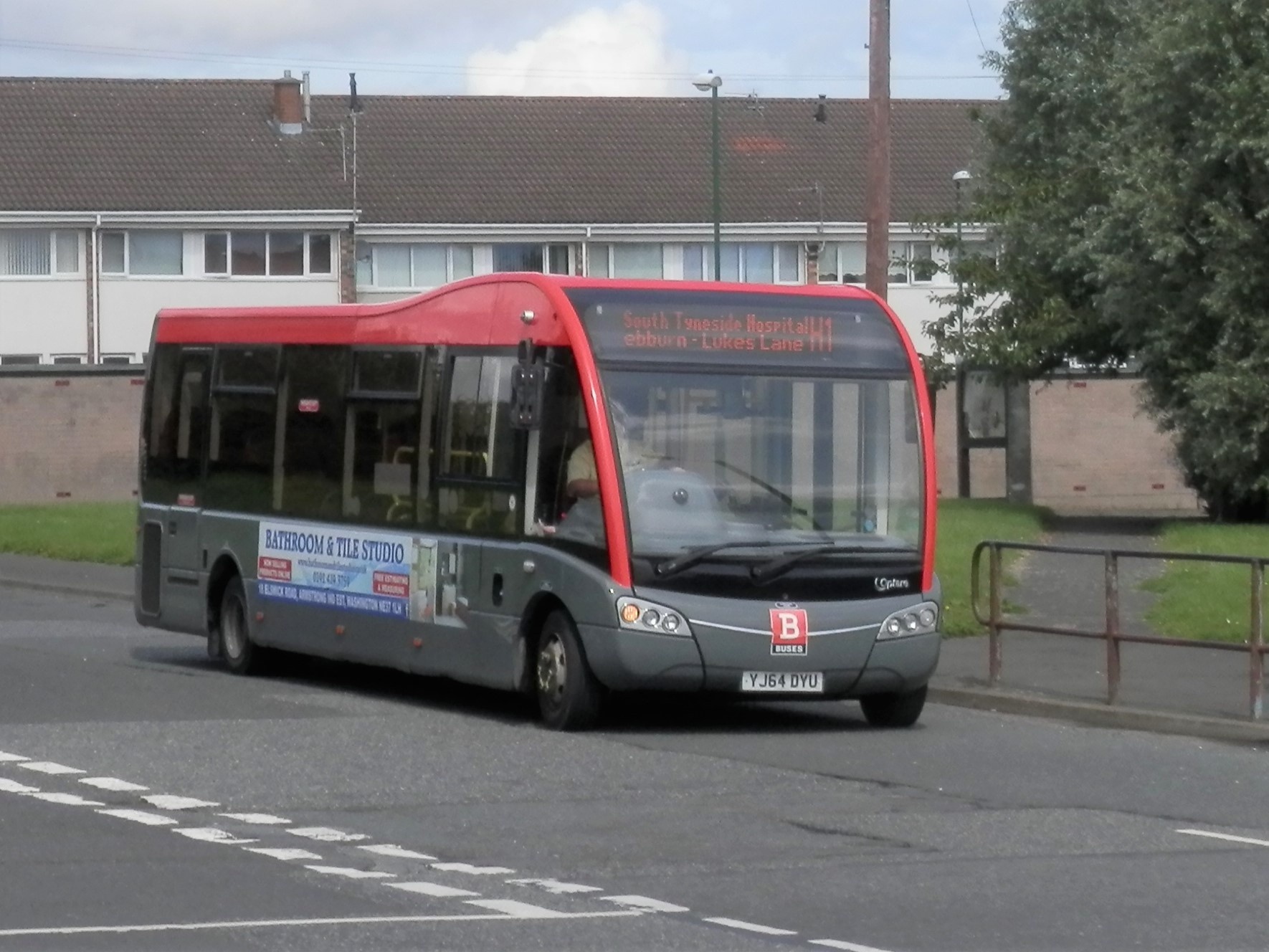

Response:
(134, 272), (944, 731)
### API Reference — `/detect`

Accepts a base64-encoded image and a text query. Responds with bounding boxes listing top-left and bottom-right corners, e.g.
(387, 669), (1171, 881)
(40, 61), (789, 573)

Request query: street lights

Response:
(953, 170), (974, 356)
(693, 70), (723, 280)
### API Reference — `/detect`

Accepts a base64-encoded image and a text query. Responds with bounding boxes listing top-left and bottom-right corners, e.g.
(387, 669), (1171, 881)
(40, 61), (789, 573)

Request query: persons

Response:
(565, 413), (685, 498)
